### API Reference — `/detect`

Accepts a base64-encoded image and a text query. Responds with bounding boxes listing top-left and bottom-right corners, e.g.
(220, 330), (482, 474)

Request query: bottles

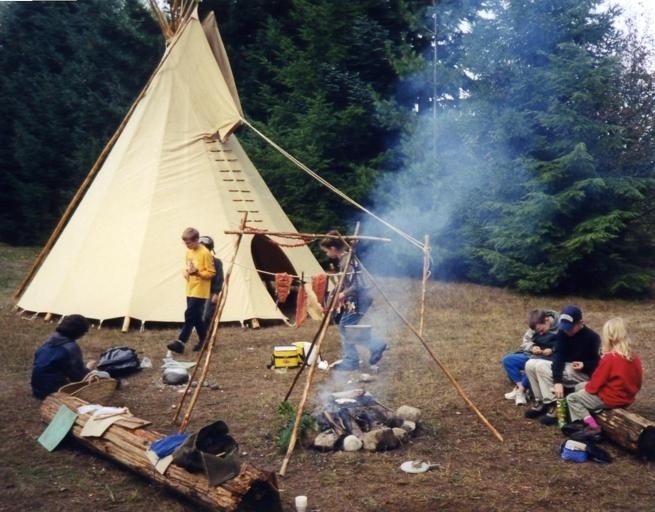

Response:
(295, 496), (308, 512)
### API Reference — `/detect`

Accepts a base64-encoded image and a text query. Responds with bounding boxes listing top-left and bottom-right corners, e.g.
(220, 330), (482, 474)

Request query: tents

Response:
(16, 7), (334, 323)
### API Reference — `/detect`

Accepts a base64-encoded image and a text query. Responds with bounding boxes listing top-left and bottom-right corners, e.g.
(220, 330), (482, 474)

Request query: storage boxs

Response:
(273, 345), (299, 369)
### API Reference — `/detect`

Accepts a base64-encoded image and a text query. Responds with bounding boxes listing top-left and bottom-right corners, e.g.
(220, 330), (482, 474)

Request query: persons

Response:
(320, 229), (387, 370)
(503, 310), (562, 405)
(567, 317), (643, 442)
(195, 238), (225, 341)
(526, 306), (602, 425)
(27, 313), (100, 396)
(168, 228), (217, 352)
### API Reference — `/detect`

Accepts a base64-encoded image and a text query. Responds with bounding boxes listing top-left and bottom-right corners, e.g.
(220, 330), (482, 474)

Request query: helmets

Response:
(199, 236), (213, 250)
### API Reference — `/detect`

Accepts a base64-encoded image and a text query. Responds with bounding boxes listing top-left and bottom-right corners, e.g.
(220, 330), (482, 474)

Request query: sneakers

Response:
(369, 343), (387, 364)
(561, 422), (588, 434)
(515, 390), (527, 406)
(504, 387), (518, 400)
(539, 406), (558, 424)
(166, 341), (186, 353)
(334, 359), (360, 372)
(524, 404), (544, 419)
(569, 425), (603, 443)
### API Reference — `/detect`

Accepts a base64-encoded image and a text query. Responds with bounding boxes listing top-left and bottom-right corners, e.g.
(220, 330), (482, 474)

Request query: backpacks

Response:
(97, 346), (141, 376)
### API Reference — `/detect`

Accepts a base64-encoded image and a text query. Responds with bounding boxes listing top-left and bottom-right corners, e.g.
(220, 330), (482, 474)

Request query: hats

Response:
(558, 305), (582, 328)
